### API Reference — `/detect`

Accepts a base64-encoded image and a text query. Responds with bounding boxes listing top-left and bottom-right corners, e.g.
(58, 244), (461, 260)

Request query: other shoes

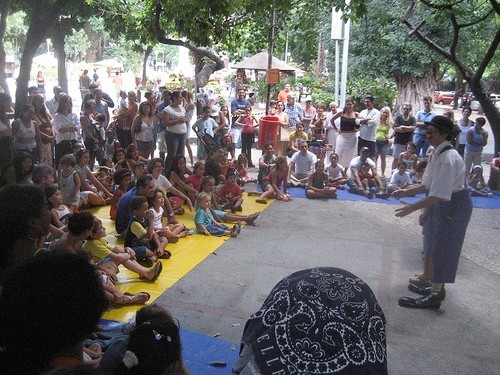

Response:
(363, 189), (373, 199)
(375, 188), (390, 199)
(246, 211), (260, 225)
(231, 220), (242, 239)
(396, 282), (446, 309)
(408, 271), (433, 284)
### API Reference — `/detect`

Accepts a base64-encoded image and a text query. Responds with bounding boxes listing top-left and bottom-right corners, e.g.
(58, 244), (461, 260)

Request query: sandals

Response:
(124, 291), (150, 305)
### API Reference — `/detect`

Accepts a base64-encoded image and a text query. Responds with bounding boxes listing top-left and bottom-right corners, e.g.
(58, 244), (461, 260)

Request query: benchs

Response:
(108, 67), (122, 76)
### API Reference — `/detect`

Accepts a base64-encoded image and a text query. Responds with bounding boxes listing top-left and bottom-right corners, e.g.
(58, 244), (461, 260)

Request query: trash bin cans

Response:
(257, 114), (279, 148)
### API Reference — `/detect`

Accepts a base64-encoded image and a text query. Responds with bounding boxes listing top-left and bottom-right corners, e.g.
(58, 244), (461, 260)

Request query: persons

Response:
(389, 114), (474, 310)
(0, 248), (106, 375)
(82, 343), (105, 364)
(0, 67), (500, 268)
(47, 211), (150, 306)
(116, 317), (191, 375)
(0, 183), (52, 275)
(98, 304), (174, 375)
(408, 159), (434, 287)
(81, 217), (163, 282)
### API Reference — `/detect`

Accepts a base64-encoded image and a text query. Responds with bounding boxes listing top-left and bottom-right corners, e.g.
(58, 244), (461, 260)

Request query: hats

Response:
(424, 115), (454, 132)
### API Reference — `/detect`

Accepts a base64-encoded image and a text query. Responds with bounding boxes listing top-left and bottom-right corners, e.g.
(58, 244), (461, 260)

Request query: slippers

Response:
(150, 261), (163, 283)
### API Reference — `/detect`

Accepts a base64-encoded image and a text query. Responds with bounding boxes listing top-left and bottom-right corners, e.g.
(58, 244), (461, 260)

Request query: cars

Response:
(435, 91), (455, 105)
(450, 90), (500, 111)
